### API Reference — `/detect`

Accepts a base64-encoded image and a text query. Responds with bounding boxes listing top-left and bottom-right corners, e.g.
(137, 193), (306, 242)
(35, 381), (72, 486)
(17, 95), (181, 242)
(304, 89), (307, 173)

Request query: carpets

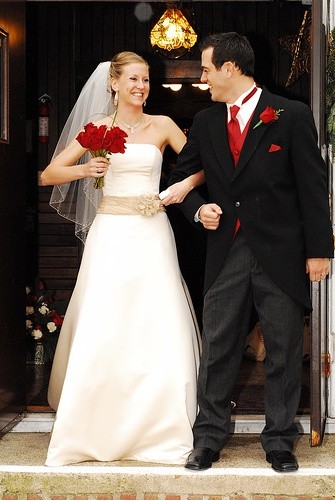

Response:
(29, 382), (310, 414)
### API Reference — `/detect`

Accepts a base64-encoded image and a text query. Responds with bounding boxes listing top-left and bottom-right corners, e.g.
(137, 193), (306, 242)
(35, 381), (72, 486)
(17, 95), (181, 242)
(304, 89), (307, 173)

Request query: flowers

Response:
(252, 106), (285, 130)
(73, 122), (128, 190)
(26, 278), (63, 340)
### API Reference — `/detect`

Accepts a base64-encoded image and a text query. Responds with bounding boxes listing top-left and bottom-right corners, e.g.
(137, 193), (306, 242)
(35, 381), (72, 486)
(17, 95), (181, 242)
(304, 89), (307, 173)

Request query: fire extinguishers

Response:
(38, 93), (52, 143)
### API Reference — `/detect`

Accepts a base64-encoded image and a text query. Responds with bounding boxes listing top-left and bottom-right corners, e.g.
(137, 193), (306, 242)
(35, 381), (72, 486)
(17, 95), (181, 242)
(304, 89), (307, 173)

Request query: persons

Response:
(40, 52), (203, 469)
(171, 32), (334, 473)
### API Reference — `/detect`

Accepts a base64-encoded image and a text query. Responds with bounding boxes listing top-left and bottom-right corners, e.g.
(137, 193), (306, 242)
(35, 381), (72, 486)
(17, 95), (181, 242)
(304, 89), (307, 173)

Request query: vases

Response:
(28, 339), (56, 380)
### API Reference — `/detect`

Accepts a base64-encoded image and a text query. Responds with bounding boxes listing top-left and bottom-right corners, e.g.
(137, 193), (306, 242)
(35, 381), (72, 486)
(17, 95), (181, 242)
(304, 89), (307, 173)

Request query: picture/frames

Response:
(0, 26), (12, 144)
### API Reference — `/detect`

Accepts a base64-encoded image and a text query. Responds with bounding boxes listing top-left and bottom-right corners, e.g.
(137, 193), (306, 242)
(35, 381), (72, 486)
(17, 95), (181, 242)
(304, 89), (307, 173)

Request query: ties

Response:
(227, 88), (259, 235)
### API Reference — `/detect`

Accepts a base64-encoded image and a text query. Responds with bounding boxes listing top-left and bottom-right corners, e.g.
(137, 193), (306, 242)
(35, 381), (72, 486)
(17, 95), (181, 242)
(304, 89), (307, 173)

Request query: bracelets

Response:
(198, 208), (202, 222)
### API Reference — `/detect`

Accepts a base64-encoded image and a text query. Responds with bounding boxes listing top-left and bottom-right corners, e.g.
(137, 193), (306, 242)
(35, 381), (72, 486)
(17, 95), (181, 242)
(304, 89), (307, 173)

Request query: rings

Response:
(321, 273), (325, 278)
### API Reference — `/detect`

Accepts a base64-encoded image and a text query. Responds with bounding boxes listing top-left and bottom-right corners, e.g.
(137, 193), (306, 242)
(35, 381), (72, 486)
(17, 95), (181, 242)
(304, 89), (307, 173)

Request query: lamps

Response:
(148, 2), (199, 61)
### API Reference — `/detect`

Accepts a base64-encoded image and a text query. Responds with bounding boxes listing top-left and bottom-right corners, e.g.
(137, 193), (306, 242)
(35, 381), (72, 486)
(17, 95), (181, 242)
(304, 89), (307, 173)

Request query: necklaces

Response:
(114, 109), (142, 133)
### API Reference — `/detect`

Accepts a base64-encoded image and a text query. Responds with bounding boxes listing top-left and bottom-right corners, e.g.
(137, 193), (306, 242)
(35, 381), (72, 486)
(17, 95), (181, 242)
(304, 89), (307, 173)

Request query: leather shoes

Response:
(266, 451), (298, 471)
(183, 447), (220, 470)
